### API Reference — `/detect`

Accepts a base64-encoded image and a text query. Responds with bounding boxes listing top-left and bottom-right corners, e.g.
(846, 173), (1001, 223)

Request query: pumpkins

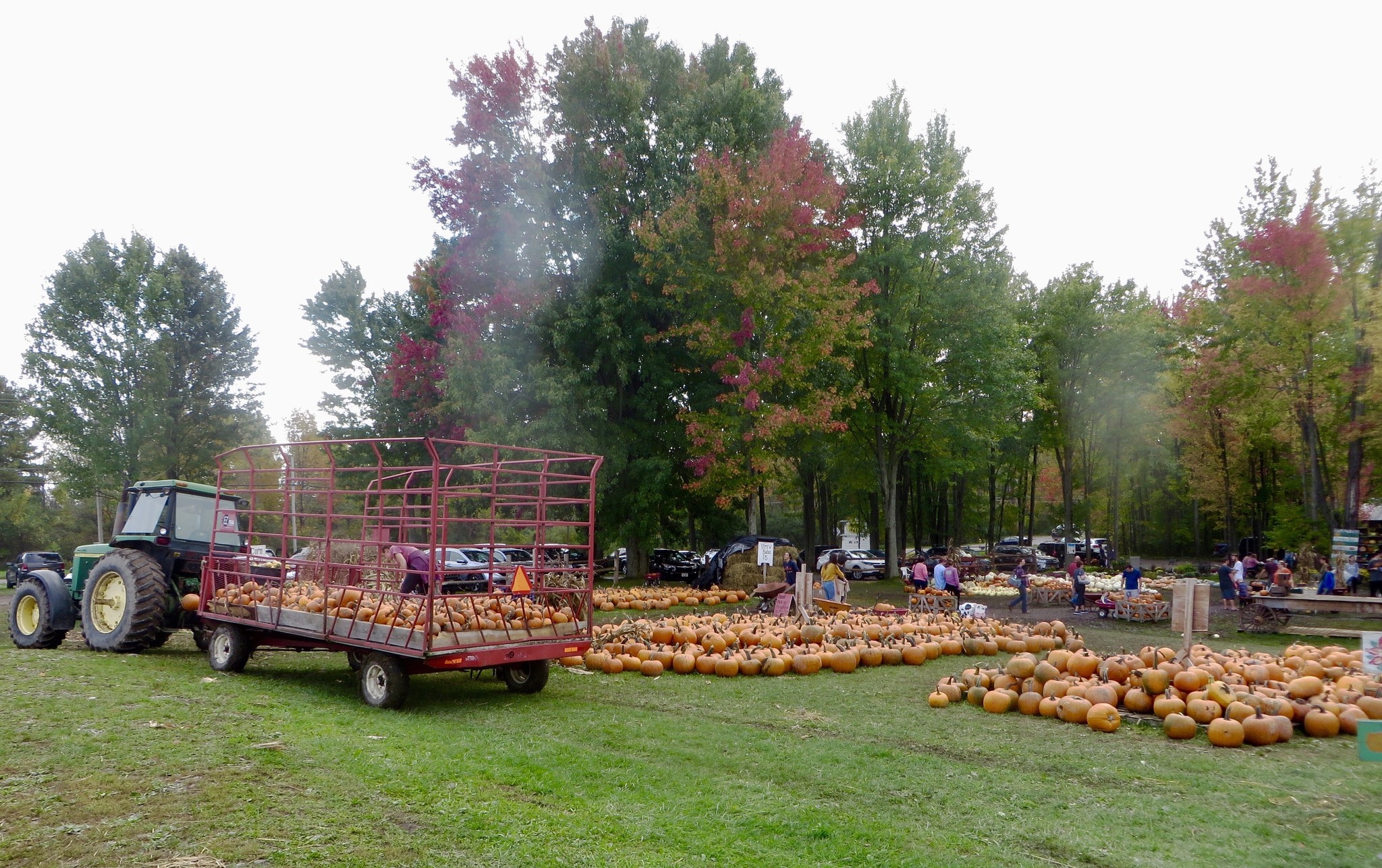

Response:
(1251, 581), (1263, 586)
(904, 571), (1221, 619)
(927, 641), (1382, 745)
(182, 561), (580, 639)
(1260, 587), (1268, 596)
(557, 582), (1084, 677)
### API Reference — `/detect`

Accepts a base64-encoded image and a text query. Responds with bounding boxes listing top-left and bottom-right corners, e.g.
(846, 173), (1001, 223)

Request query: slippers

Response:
(1224, 607), (1229, 610)
(1232, 608), (1240, 610)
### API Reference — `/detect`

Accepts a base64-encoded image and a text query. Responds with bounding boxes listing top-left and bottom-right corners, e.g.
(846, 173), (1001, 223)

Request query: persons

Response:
(1067, 556), (1089, 615)
(944, 559), (961, 610)
(934, 557), (947, 591)
(912, 556), (928, 593)
(499, 588), (535, 605)
(821, 553), (850, 603)
(1008, 558), (1031, 613)
(1121, 564), (1142, 600)
(63, 567), (73, 579)
(1219, 550), (1382, 611)
(382, 545), (441, 601)
(1098, 544), (1106, 567)
(783, 552), (800, 597)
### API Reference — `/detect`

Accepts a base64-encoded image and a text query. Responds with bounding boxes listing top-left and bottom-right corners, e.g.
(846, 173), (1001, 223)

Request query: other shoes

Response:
(1023, 610), (1031, 613)
(1081, 610), (1088, 613)
(1069, 601), (1076, 609)
(1007, 604), (1012, 612)
(1074, 611), (1082, 615)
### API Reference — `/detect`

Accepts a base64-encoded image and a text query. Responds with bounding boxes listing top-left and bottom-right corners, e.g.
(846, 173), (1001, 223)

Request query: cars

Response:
(421, 544), (600, 592)
(654, 548), (699, 582)
(926, 546), (996, 571)
(672, 550), (702, 566)
(264, 547), (312, 578)
(869, 550), (902, 567)
(994, 535), (1028, 546)
(1052, 523), (1080, 541)
(700, 548), (721, 567)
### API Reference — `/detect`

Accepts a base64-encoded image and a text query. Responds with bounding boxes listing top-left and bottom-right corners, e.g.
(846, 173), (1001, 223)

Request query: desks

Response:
(1250, 592), (1382, 614)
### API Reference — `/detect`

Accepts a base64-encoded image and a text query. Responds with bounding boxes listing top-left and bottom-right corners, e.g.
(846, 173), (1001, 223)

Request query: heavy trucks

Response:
(7, 434), (604, 711)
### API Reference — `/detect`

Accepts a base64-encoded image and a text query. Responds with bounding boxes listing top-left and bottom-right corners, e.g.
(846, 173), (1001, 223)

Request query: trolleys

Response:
(752, 580), (796, 612)
(812, 597), (868, 617)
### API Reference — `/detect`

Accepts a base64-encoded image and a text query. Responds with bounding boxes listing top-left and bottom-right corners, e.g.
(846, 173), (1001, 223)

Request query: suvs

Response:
(1079, 538), (1114, 558)
(1037, 542), (1101, 566)
(816, 548), (886, 581)
(602, 547), (656, 575)
(6, 550), (66, 589)
(988, 545), (1060, 573)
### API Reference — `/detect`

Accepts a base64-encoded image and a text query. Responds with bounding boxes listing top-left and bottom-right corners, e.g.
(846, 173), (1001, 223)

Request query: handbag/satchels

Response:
(1007, 568), (1022, 588)
(909, 564), (917, 583)
(1077, 569), (1090, 584)
